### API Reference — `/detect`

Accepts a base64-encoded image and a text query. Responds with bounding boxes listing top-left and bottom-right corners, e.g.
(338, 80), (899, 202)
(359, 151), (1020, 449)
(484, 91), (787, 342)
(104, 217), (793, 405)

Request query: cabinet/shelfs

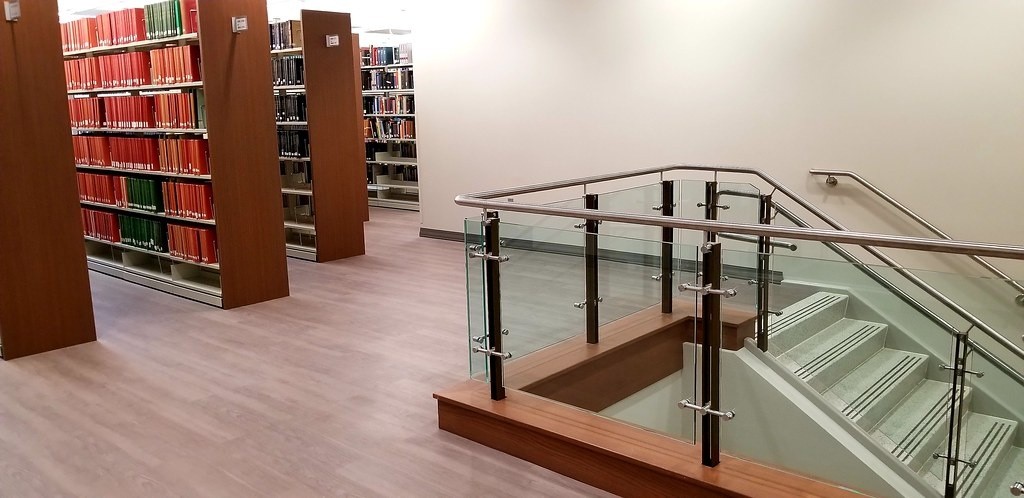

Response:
(0, 0), (419, 359)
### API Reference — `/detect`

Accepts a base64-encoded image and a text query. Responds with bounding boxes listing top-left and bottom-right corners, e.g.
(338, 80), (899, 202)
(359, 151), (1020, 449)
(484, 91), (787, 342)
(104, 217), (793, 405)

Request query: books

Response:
(269, 20), (317, 227)
(63, 0), (218, 264)
(359, 42), (418, 184)
